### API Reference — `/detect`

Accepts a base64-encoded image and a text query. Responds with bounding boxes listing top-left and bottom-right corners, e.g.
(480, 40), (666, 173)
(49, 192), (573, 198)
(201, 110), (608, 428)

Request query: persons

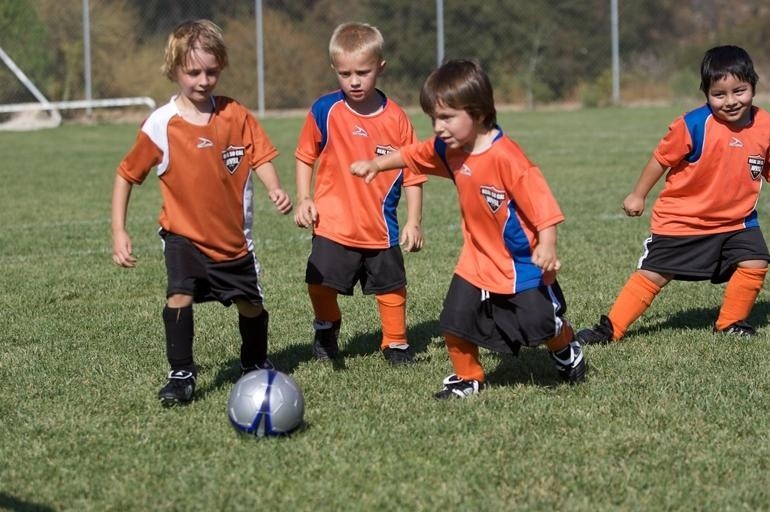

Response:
(110, 19), (294, 408)
(574, 44), (770, 347)
(351, 59), (586, 399)
(294, 20), (427, 368)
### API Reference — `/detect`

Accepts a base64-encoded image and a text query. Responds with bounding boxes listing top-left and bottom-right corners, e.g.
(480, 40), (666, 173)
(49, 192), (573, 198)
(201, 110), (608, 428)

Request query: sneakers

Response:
(239, 357), (276, 378)
(158, 365), (198, 408)
(311, 317), (349, 360)
(712, 318), (762, 338)
(575, 314), (615, 347)
(547, 334), (587, 387)
(378, 342), (419, 370)
(433, 372), (488, 401)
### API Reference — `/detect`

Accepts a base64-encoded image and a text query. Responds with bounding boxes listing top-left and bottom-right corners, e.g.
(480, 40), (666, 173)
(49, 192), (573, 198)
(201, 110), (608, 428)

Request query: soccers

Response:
(228, 370), (304, 441)
